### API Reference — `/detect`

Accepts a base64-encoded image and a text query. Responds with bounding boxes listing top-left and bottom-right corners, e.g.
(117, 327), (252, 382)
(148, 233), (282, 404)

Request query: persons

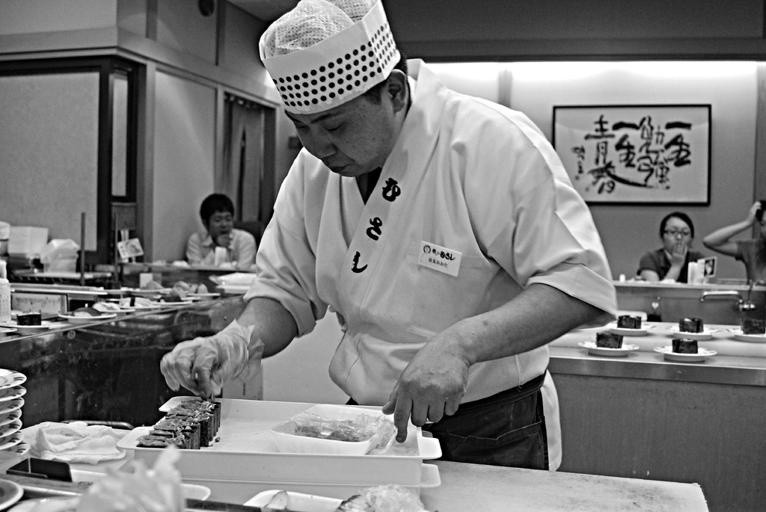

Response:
(702, 200), (766, 285)
(158, 0), (618, 470)
(185, 192), (256, 269)
(635, 211), (705, 285)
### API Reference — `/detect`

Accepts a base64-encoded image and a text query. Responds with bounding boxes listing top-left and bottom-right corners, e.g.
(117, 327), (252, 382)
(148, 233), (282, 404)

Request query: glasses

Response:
(664, 230), (690, 237)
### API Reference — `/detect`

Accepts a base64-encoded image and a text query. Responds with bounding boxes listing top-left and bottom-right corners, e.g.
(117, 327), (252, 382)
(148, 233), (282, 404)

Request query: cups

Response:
(679, 316), (704, 333)
(595, 332), (623, 350)
(616, 314), (643, 331)
(15, 311), (43, 327)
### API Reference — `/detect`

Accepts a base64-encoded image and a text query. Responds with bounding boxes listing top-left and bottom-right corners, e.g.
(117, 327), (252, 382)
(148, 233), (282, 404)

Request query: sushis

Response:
(138, 399), (222, 450)
(596, 332), (622, 348)
(680, 317), (703, 333)
(742, 319), (765, 334)
(617, 315), (640, 328)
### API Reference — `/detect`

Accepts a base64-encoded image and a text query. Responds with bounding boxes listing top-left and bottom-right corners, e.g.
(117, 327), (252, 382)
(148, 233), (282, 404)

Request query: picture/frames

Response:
(552, 102), (713, 210)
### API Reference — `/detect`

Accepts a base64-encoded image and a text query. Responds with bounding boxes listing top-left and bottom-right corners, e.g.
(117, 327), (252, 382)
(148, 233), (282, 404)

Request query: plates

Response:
(0, 361), (29, 511)
(576, 340), (639, 357)
(0, 323), (59, 338)
(609, 323), (653, 337)
(667, 327), (716, 340)
(651, 344), (717, 363)
(53, 303), (163, 321)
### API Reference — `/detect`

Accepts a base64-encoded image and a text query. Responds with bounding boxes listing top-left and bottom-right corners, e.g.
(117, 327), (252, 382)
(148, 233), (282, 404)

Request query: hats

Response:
(259, 0), (401, 114)
